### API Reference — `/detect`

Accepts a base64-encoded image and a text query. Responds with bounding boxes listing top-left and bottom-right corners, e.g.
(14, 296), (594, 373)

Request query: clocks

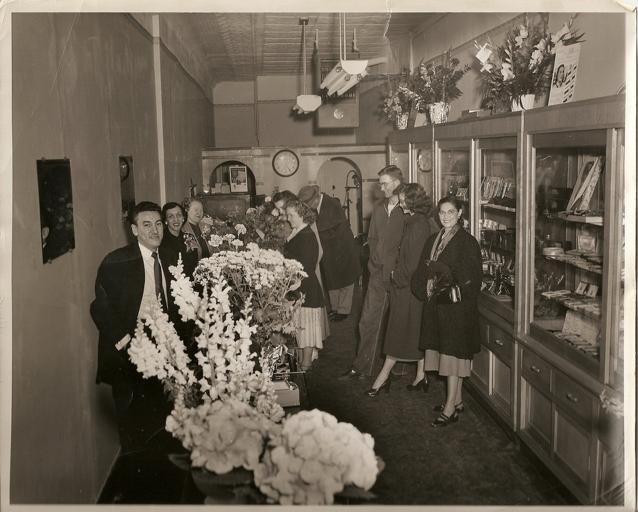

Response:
(272, 150), (299, 177)
(120, 158), (129, 181)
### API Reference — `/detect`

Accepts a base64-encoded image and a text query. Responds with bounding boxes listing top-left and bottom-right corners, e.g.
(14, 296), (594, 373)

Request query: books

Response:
(563, 156), (604, 216)
(480, 177), (510, 204)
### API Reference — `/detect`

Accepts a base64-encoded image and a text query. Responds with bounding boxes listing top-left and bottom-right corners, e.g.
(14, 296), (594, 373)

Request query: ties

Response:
(152, 252), (167, 313)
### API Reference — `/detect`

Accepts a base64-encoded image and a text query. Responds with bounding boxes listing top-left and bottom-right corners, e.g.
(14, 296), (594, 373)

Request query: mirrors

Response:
(119, 156), (135, 220)
(36, 157), (75, 264)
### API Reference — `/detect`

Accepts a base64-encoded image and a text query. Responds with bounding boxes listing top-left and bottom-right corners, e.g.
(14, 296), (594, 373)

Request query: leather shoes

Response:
(338, 367), (365, 380)
(432, 401), (464, 426)
(329, 311), (348, 321)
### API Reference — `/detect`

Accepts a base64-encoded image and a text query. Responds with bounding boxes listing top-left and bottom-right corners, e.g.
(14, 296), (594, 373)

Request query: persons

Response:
(159, 202), (197, 332)
(410, 194), (483, 428)
(266, 185), (358, 372)
(365, 183), (430, 397)
(89, 201), (173, 493)
(552, 63), (567, 88)
(339, 164), (406, 381)
(180, 198), (212, 264)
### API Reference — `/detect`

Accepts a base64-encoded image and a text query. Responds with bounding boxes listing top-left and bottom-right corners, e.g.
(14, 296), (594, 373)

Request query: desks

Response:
(202, 194), (265, 218)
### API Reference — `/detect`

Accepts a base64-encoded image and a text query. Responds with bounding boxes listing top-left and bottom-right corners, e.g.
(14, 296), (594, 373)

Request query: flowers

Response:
(376, 13), (585, 120)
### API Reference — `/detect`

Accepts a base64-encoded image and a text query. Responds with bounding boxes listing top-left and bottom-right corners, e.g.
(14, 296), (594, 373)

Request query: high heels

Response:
(407, 375), (429, 391)
(366, 378), (390, 397)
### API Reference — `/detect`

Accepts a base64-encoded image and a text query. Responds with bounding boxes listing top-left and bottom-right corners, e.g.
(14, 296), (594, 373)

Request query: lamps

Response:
(292, 12), (368, 116)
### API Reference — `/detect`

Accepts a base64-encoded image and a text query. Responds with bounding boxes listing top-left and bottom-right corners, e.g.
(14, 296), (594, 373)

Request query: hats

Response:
(299, 185), (319, 207)
